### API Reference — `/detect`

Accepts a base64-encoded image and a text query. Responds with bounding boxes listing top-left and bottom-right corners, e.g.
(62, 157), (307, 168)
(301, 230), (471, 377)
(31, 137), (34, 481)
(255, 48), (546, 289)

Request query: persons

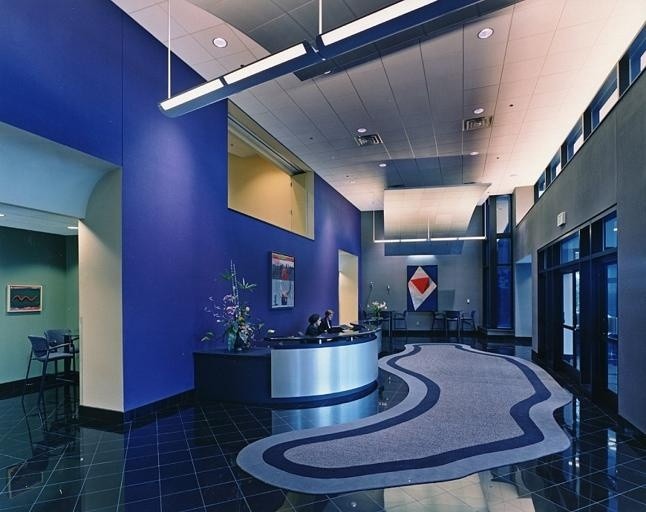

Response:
(304, 313), (321, 337)
(318, 309), (334, 332)
(279, 268), (288, 281)
(280, 292), (288, 305)
